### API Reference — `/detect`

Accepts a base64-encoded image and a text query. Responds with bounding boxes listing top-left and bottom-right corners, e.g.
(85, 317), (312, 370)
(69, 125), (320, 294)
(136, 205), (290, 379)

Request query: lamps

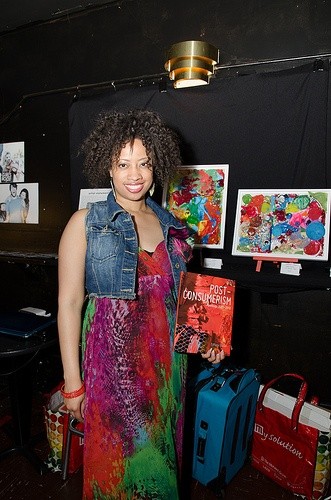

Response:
(162, 40), (220, 90)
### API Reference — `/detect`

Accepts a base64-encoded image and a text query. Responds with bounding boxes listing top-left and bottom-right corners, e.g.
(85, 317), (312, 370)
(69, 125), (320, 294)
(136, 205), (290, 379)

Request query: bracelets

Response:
(61, 383), (85, 399)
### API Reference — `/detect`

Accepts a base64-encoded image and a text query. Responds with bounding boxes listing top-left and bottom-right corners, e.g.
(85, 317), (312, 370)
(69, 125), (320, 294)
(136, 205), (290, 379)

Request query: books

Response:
(174, 271), (235, 356)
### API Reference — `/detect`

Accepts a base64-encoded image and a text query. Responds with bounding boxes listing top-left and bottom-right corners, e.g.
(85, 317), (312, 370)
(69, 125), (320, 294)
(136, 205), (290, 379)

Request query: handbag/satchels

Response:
(187, 363), (260, 492)
(44, 379), (84, 476)
(253, 372), (331, 500)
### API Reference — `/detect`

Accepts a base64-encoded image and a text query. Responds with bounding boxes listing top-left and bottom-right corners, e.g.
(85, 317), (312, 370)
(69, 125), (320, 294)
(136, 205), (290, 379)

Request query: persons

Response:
(0, 152), (30, 223)
(58, 106), (225, 500)
